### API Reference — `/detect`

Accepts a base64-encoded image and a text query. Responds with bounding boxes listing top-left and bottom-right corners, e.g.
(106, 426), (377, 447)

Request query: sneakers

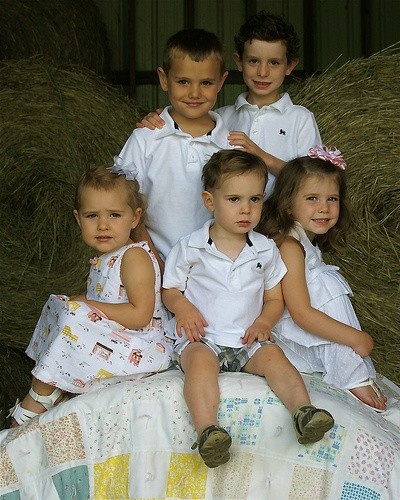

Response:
(191, 424), (232, 469)
(293, 406), (334, 444)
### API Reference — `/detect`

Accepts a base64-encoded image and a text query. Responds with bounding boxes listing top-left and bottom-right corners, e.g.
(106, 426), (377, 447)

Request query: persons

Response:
(113, 26), (246, 276)
(8, 165), (175, 428)
(136, 15), (323, 205)
(255, 153), (391, 413)
(161, 149), (334, 468)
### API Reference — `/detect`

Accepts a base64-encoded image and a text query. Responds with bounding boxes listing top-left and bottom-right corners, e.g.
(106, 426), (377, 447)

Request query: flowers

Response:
(308, 144), (348, 171)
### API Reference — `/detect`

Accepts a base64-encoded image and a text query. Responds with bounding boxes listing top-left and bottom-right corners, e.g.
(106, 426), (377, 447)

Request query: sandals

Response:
(7, 388), (69, 429)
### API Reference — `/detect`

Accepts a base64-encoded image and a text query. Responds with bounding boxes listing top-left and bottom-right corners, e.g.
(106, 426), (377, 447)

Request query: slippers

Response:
(342, 379), (388, 412)
